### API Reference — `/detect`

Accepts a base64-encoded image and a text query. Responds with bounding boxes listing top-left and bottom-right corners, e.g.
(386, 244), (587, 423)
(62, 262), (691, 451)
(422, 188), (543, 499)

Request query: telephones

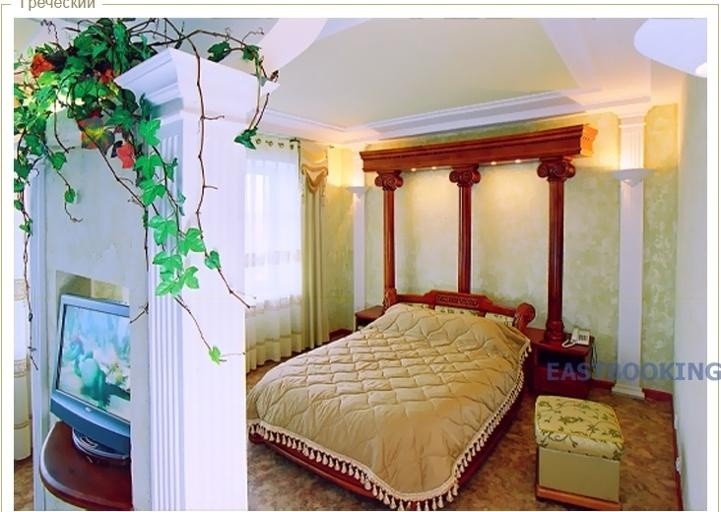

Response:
(570, 327), (590, 346)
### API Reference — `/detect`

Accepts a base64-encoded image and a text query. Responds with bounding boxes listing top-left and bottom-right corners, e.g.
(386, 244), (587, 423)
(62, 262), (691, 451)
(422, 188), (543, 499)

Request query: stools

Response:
(534, 394), (623, 510)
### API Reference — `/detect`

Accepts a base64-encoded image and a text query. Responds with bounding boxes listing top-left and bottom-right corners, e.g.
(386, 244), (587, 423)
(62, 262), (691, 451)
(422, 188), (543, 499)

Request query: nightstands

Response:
(530, 330), (595, 401)
(354, 305), (383, 334)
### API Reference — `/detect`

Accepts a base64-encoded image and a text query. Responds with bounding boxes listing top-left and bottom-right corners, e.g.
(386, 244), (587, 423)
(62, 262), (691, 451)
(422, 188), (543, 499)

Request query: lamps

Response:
(610, 169), (658, 187)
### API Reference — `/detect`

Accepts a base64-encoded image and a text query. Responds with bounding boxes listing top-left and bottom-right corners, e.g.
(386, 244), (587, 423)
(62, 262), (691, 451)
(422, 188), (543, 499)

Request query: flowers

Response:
(13, 16), (279, 373)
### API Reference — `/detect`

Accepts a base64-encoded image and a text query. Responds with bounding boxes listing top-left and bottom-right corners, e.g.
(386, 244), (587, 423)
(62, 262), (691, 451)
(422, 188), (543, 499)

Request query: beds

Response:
(245, 288), (535, 507)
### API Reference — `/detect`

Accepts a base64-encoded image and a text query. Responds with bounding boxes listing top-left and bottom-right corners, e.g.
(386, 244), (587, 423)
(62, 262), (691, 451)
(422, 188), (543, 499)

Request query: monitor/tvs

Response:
(49, 292), (130, 460)
